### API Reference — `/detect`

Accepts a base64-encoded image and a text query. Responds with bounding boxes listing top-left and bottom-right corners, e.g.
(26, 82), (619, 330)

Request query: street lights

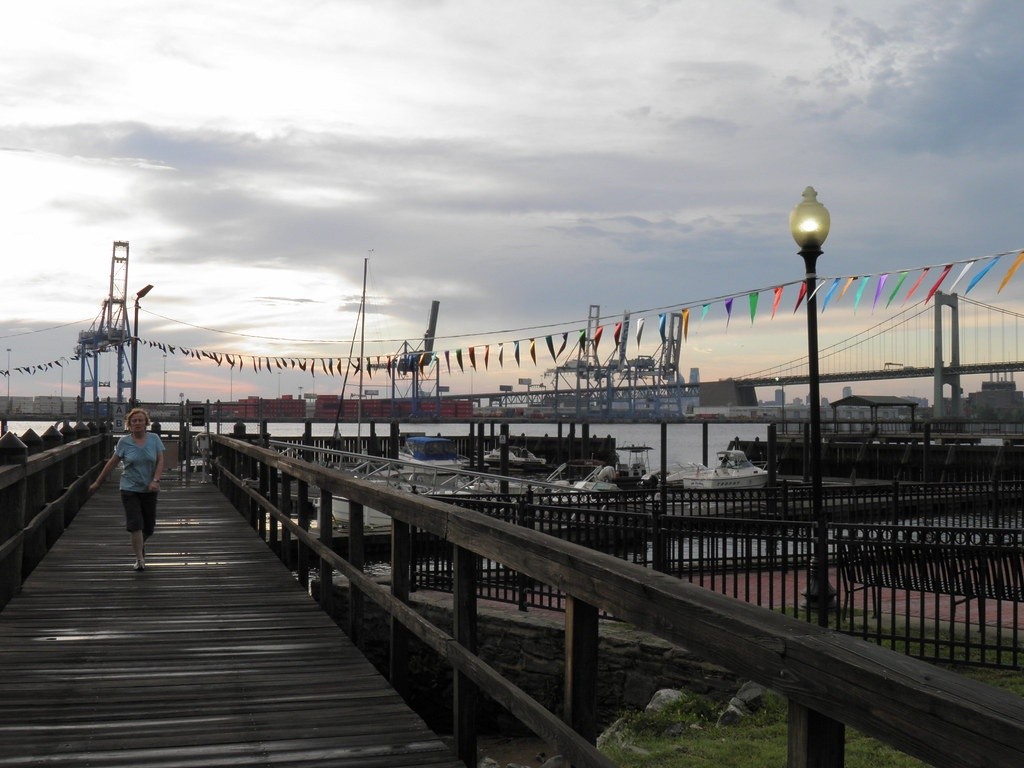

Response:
(469, 366), (475, 395)
(787, 184), (847, 614)
(59, 357), (66, 413)
(277, 371), (282, 400)
(127, 284), (154, 415)
(161, 355), (167, 404)
(6, 348), (12, 415)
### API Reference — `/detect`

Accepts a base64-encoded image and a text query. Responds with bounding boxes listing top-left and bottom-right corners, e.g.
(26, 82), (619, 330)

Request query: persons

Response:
(89, 408), (166, 570)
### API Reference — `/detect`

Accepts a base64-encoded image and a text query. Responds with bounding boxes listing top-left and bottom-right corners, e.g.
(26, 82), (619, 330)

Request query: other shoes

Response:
(134, 555), (147, 569)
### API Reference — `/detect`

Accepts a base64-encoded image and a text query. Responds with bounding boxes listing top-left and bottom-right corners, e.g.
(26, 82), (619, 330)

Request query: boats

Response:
(264, 435), (767, 534)
